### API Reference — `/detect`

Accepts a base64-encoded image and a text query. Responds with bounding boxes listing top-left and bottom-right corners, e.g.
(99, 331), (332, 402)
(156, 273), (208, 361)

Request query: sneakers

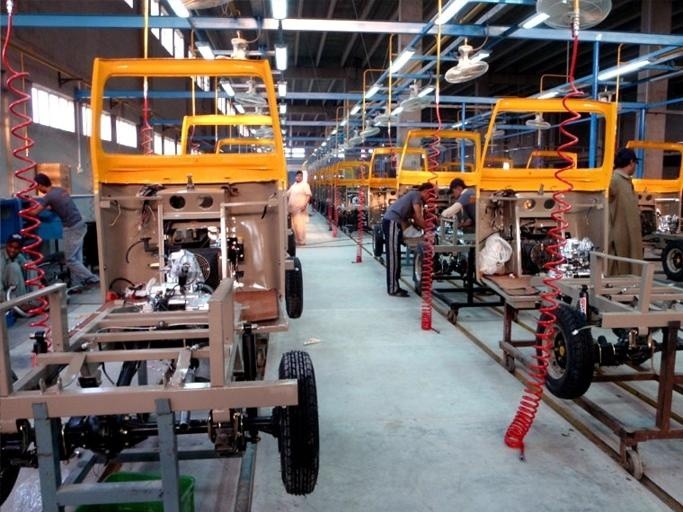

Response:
(85, 280), (101, 289)
(393, 287), (409, 293)
(389, 291), (410, 297)
(67, 289), (83, 295)
(296, 241), (306, 246)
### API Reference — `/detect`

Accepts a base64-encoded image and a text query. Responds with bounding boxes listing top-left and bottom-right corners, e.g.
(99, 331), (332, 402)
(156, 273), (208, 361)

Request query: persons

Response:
(30, 176), (98, 293)
(382, 183), (434, 297)
(284, 169), (314, 246)
(441, 178), (474, 230)
(0, 231), (48, 315)
(611, 160), (643, 277)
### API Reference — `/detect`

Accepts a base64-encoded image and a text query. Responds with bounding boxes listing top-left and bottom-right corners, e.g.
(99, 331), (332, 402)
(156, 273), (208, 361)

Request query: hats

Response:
(446, 178), (465, 195)
(420, 182), (439, 199)
(614, 147), (645, 166)
(6, 232), (25, 245)
(29, 173), (46, 188)
